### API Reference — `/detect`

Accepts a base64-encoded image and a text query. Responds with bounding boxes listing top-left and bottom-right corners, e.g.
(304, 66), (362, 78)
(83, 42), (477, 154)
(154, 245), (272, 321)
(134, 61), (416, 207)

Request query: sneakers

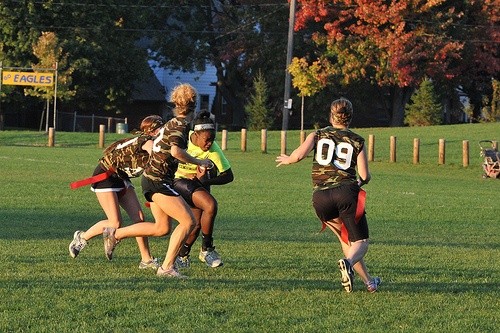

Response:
(69, 230), (89, 258)
(103, 227), (121, 260)
(338, 259), (355, 293)
(175, 255), (191, 269)
(138, 258), (161, 269)
(199, 246), (223, 267)
(156, 265), (188, 278)
(367, 277), (382, 293)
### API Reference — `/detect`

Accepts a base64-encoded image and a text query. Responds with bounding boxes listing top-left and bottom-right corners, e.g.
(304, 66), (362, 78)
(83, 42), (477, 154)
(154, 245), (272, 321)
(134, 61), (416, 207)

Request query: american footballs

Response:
(199, 161), (217, 179)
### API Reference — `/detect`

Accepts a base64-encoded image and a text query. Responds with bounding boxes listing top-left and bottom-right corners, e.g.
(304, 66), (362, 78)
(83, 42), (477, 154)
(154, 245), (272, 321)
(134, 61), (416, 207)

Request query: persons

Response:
(69, 115), (164, 270)
(103, 83), (213, 279)
(174, 109), (234, 268)
(275, 99), (382, 293)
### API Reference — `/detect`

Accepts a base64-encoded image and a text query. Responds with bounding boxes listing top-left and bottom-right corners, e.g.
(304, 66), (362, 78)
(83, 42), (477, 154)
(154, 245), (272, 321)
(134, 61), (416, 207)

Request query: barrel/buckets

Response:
(116, 123), (128, 134)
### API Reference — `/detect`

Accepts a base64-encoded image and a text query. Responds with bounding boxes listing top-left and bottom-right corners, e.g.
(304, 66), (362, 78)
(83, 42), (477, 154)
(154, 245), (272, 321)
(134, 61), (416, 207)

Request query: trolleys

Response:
(479, 139), (500, 178)
